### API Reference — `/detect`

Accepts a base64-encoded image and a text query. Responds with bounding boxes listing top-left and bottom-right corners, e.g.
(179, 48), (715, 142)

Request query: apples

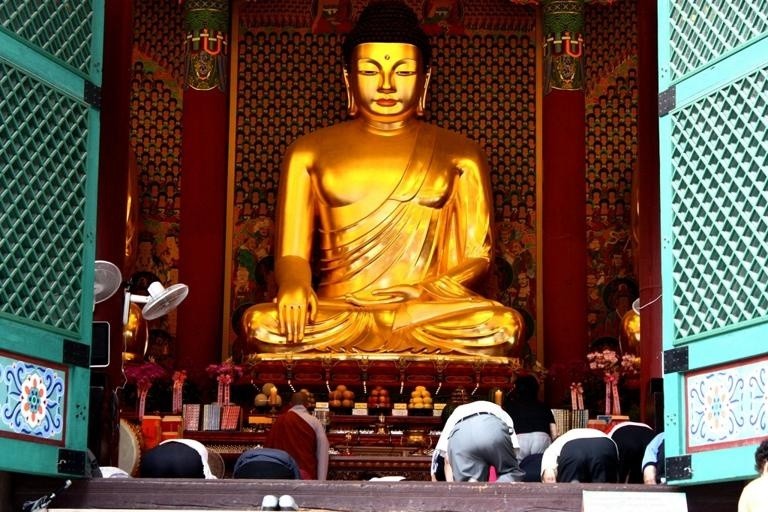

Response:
(367, 387), (391, 408)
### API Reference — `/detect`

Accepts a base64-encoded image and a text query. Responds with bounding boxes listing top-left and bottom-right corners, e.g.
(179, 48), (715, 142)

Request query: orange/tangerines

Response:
(407, 385), (433, 408)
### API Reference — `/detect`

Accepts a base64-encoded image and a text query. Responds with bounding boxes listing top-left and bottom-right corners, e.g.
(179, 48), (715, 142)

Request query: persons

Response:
(636, 430), (667, 485)
(429, 399), (528, 482)
(735, 437), (768, 512)
(232, 446), (300, 480)
(497, 373), (559, 465)
(139, 437), (218, 479)
(265, 391), (330, 479)
(539, 427), (621, 484)
(607, 420), (653, 482)
(238, 0), (527, 353)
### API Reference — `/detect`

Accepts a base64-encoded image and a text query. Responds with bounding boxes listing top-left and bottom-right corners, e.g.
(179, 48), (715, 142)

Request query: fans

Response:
(123, 282), (189, 325)
(89, 261), (122, 314)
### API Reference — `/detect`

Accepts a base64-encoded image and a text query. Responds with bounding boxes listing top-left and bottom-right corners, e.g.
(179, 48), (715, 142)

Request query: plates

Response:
(256, 404), (433, 418)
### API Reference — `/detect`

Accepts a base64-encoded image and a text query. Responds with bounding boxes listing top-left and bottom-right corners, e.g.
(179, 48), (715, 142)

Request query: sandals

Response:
(261, 494), (299, 511)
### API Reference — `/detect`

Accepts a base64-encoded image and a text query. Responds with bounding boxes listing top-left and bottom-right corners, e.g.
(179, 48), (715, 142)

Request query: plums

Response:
(451, 384), (470, 404)
(301, 389), (316, 408)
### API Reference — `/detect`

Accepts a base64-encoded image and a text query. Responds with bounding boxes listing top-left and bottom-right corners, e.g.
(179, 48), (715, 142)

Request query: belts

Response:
(455, 412), (494, 425)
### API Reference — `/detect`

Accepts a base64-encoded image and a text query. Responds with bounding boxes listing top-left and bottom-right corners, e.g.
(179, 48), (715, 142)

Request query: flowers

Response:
(125, 361), (166, 390)
(171, 368), (188, 381)
(205, 356), (234, 376)
(586, 349), (641, 382)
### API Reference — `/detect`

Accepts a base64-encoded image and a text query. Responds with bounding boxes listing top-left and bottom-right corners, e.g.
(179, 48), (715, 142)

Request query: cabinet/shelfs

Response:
(182, 412), (441, 481)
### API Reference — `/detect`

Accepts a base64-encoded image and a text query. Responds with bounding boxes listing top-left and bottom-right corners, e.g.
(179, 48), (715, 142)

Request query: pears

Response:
(254, 383), (282, 406)
